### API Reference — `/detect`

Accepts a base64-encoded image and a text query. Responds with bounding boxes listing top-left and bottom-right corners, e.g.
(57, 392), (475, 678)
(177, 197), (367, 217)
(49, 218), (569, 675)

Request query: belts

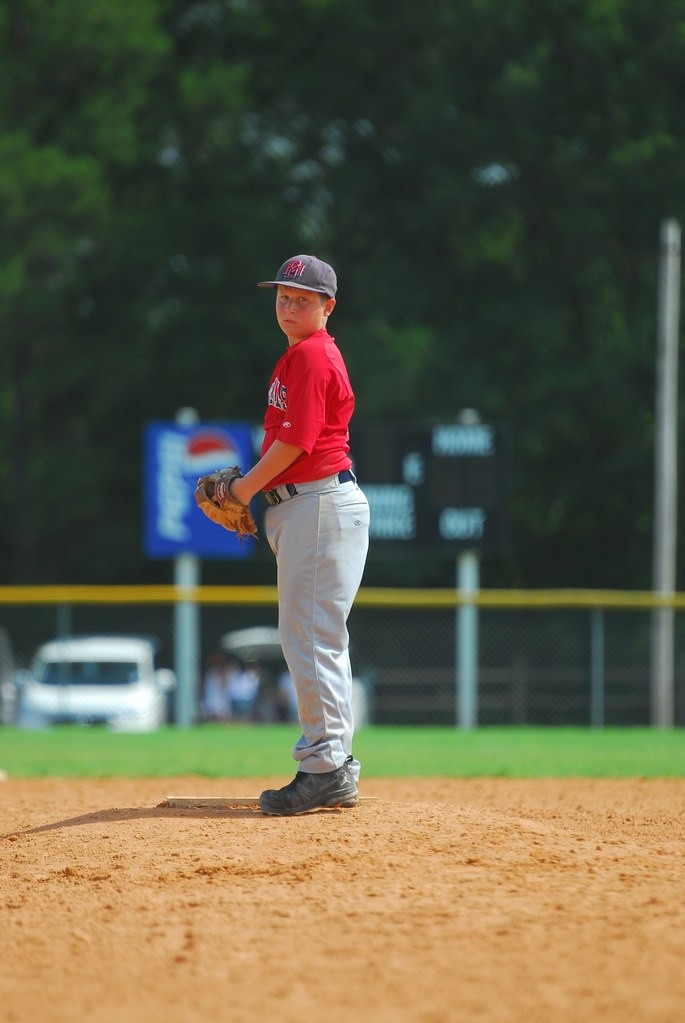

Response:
(264, 470), (356, 506)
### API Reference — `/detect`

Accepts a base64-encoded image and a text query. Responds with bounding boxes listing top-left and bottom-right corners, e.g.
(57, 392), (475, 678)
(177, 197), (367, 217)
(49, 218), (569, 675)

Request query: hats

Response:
(257, 254), (337, 298)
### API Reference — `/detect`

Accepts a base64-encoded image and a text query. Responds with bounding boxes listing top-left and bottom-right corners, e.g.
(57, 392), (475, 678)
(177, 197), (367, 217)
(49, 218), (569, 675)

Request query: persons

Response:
(206, 255), (372, 813)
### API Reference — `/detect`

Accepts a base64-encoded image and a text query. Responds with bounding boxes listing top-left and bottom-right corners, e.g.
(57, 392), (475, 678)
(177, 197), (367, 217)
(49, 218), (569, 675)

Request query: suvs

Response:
(193, 624), (373, 729)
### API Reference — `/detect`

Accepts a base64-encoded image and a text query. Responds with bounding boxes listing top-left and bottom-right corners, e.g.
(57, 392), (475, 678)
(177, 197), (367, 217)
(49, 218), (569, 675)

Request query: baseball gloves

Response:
(194, 465), (260, 542)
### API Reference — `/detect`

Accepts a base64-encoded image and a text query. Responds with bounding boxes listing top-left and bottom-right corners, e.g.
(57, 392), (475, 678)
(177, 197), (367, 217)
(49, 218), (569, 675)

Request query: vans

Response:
(14, 635), (173, 733)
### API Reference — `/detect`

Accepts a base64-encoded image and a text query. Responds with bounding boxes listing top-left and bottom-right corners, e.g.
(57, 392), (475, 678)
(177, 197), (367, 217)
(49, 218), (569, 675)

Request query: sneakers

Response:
(259, 773), (361, 815)
